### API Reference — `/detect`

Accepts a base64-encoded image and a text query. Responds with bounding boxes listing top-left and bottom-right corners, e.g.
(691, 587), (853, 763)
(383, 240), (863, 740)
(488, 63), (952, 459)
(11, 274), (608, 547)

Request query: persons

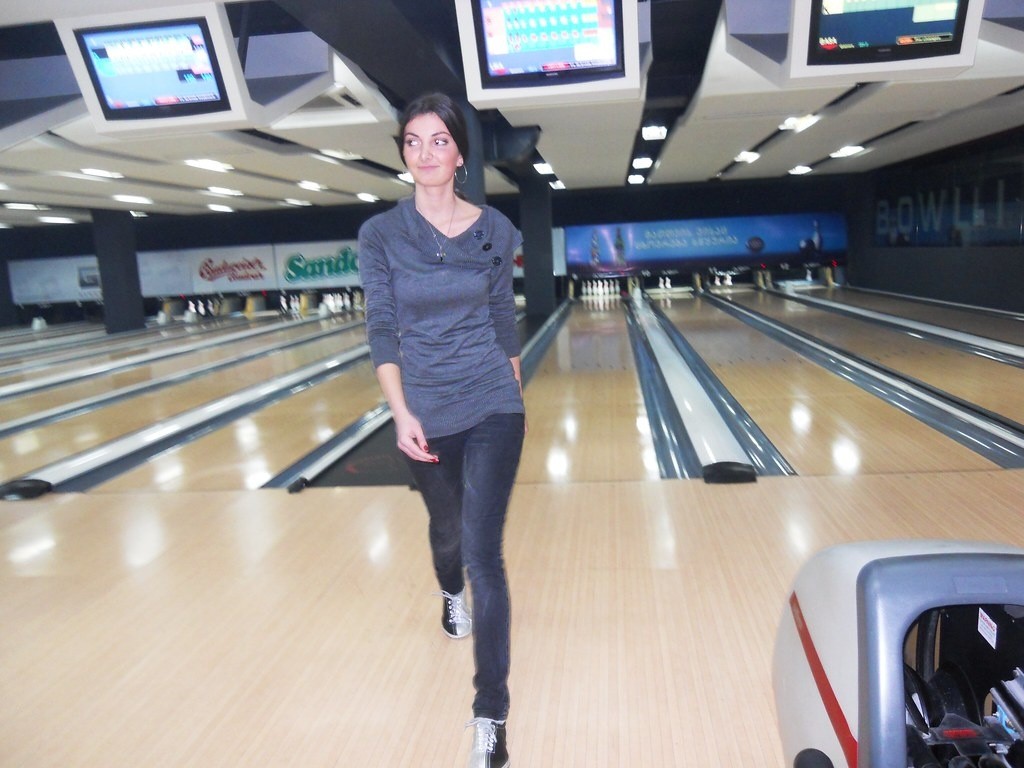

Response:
(358, 92), (528, 768)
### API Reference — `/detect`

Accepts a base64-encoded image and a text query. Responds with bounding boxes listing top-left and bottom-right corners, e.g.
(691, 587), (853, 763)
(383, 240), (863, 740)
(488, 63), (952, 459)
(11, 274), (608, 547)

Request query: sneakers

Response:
(465, 717), (511, 768)
(431, 586), (473, 639)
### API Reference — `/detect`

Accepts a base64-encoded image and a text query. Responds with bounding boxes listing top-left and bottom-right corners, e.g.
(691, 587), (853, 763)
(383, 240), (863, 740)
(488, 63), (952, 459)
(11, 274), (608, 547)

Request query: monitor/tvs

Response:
(51, 3), (252, 131)
(454, 1), (640, 109)
(788, 0), (984, 88)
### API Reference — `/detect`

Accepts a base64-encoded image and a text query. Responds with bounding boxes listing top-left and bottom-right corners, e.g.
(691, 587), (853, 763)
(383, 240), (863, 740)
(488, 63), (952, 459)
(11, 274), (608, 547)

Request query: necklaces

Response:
(416, 196), (455, 262)
